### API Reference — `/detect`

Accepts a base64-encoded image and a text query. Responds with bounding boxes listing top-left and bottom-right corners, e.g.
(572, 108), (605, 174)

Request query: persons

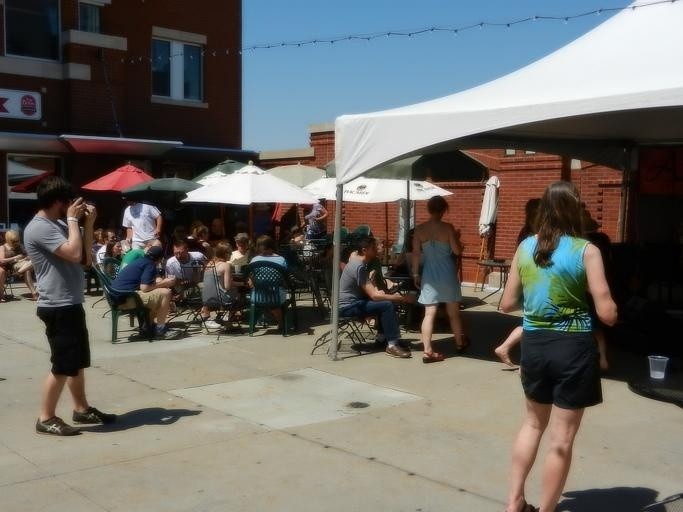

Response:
(495, 198), (612, 373)
(23, 175), (117, 437)
(499, 179), (622, 512)
(3, 194), (468, 361)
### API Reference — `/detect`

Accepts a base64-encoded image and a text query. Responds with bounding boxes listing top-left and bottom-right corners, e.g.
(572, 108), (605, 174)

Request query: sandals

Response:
(422, 351), (444, 364)
(454, 339), (469, 351)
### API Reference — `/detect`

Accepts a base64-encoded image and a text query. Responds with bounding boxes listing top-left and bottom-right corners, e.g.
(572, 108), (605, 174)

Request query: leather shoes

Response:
(384, 345), (411, 358)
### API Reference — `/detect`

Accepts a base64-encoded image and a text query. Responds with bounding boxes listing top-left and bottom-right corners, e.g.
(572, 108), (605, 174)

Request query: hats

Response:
(147, 246), (163, 258)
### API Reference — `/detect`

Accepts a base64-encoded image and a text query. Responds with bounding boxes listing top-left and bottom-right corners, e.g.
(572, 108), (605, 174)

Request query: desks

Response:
(476, 258), (513, 293)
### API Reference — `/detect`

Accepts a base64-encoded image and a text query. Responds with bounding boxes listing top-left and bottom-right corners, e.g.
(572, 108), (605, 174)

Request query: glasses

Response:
(225, 251), (231, 255)
(9, 239), (19, 244)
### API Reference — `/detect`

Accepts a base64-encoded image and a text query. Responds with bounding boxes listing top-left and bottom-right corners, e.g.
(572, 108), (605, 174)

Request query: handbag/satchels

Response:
(384, 265), (409, 278)
(383, 279), (412, 297)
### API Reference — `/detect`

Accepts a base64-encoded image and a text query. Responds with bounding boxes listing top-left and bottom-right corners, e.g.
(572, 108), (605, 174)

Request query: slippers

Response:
(504, 500), (536, 512)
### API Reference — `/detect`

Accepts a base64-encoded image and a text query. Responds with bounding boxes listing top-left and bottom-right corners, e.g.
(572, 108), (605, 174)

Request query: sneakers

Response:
(35, 417), (81, 436)
(72, 407), (117, 424)
(152, 330), (181, 340)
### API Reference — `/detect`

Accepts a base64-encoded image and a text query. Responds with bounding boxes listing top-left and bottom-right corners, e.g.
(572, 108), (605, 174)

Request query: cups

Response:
(647, 355), (668, 379)
